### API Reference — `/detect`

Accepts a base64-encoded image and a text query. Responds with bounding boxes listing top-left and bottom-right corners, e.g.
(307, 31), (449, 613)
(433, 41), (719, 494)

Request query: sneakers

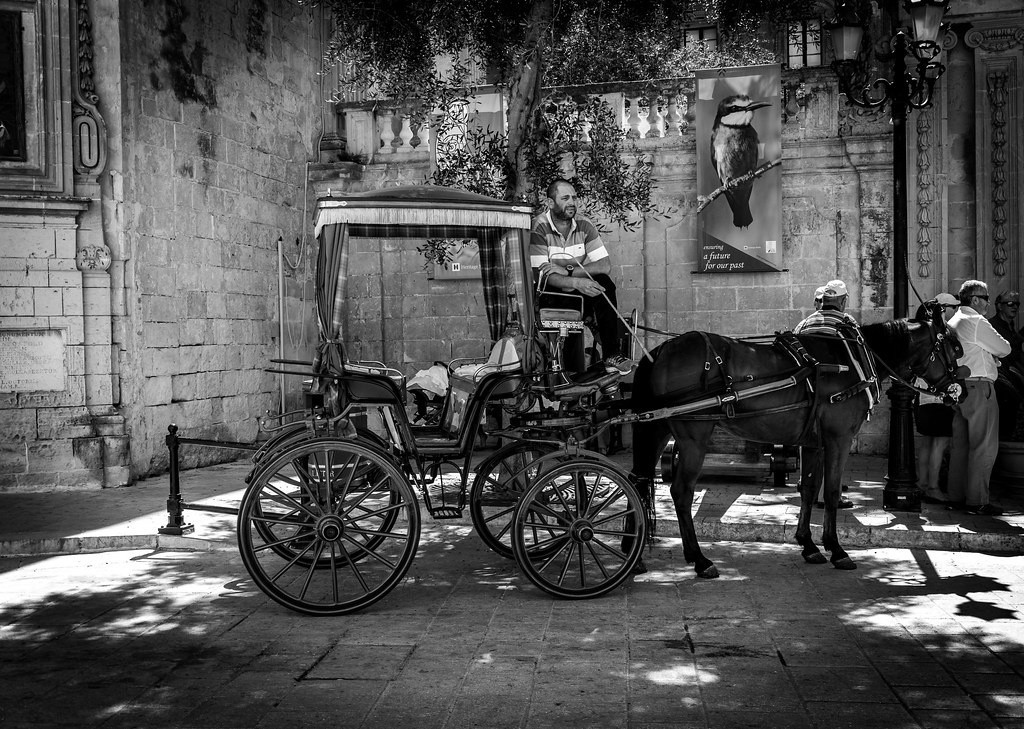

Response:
(584, 313), (602, 346)
(603, 354), (638, 375)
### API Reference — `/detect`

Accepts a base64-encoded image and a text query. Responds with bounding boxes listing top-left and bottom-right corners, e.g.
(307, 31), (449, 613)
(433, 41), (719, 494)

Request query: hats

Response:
(934, 293), (961, 305)
(822, 279), (849, 297)
(814, 286), (826, 299)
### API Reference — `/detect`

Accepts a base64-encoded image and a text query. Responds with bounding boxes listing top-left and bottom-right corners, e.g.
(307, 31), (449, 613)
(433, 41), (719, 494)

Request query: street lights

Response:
(826, 0), (945, 512)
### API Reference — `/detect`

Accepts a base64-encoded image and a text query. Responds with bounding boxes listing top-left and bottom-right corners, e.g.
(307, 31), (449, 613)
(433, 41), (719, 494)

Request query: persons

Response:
(946, 280), (1013, 518)
(801, 280), (861, 507)
(986, 290), (1024, 443)
(525, 180), (637, 377)
(914, 293), (962, 508)
(793, 286), (827, 490)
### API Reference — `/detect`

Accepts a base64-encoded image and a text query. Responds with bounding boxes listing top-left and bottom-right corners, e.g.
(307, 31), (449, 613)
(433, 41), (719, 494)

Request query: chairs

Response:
(331, 319), (414, 447)
(441, 321), (527, 440)
(661, 425), (798, 486)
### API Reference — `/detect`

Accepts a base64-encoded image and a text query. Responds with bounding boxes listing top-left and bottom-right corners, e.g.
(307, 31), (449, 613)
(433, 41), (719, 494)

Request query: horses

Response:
(621, 299), (969, 580)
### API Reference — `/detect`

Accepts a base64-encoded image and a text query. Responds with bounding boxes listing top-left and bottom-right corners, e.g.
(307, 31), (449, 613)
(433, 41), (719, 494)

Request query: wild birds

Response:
(710, 94), (773, 232)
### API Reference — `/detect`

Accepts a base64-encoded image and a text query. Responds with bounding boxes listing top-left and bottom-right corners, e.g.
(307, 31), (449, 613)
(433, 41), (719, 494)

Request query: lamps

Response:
(821, 0), (952, 112)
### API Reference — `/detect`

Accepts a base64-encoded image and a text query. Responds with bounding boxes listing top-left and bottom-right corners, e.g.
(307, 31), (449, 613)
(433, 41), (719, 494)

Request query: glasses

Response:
(996, 301), (1020, 307)
(941, 308), (946, 313)
(816, 299), (822, 303)
(970, 295), (989, 301)
(943, 305), (956, 309)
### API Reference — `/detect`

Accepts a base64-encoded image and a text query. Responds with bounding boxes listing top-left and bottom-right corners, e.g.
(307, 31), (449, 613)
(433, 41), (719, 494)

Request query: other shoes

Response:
(967, 505), (1003, 515)
(843, 486), (848, 491)
(923, 492), (952, 505)
(817, 500), (853, 507)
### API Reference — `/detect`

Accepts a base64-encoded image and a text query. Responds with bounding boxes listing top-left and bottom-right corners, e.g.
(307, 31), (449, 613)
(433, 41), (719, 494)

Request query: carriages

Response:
(235, 184), (971, 617)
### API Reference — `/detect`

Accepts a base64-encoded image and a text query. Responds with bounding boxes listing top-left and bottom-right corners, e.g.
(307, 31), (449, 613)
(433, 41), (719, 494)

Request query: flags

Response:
(691, 62), (783, 272)
